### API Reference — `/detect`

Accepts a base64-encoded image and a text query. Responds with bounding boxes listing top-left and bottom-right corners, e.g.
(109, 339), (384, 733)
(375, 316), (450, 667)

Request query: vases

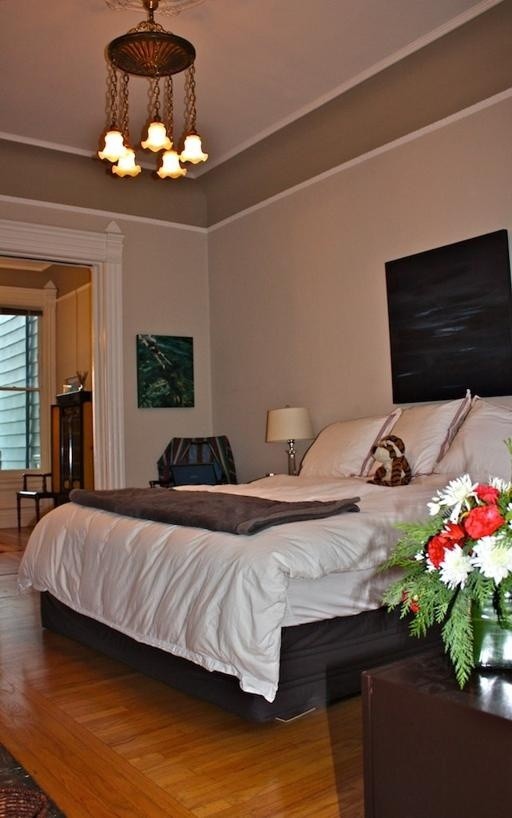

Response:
(477, 589), (512, 672)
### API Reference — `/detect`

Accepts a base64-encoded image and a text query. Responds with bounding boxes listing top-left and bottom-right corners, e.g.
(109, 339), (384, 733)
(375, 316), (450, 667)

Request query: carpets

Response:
(0, 743), (64, 817)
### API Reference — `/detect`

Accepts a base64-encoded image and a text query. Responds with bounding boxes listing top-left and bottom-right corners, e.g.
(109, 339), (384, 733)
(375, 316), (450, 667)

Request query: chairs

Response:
(148, 437), (237, 490)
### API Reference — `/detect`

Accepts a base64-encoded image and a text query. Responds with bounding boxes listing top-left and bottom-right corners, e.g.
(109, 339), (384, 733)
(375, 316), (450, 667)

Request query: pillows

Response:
(298, 391), (512, 478)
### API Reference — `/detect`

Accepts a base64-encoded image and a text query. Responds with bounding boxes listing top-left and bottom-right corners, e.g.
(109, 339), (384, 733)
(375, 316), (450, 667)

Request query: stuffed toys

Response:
(364, 435), (412, 487)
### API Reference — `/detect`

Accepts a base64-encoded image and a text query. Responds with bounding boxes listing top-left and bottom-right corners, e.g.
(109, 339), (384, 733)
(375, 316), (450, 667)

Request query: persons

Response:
(138, 334), (174, 371)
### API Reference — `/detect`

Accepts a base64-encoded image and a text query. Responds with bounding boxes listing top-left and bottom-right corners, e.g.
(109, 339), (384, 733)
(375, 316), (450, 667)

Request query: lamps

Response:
(99, 2), (206, 179)
(265, 406), (317, 477)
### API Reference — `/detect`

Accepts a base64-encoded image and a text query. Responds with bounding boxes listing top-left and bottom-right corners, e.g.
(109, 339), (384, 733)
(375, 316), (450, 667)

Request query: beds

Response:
(20, 477), (512, 722)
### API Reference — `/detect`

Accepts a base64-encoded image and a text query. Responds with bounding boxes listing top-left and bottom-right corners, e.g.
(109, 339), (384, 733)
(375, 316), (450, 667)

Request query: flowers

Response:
(375, 475), (512, 689)
